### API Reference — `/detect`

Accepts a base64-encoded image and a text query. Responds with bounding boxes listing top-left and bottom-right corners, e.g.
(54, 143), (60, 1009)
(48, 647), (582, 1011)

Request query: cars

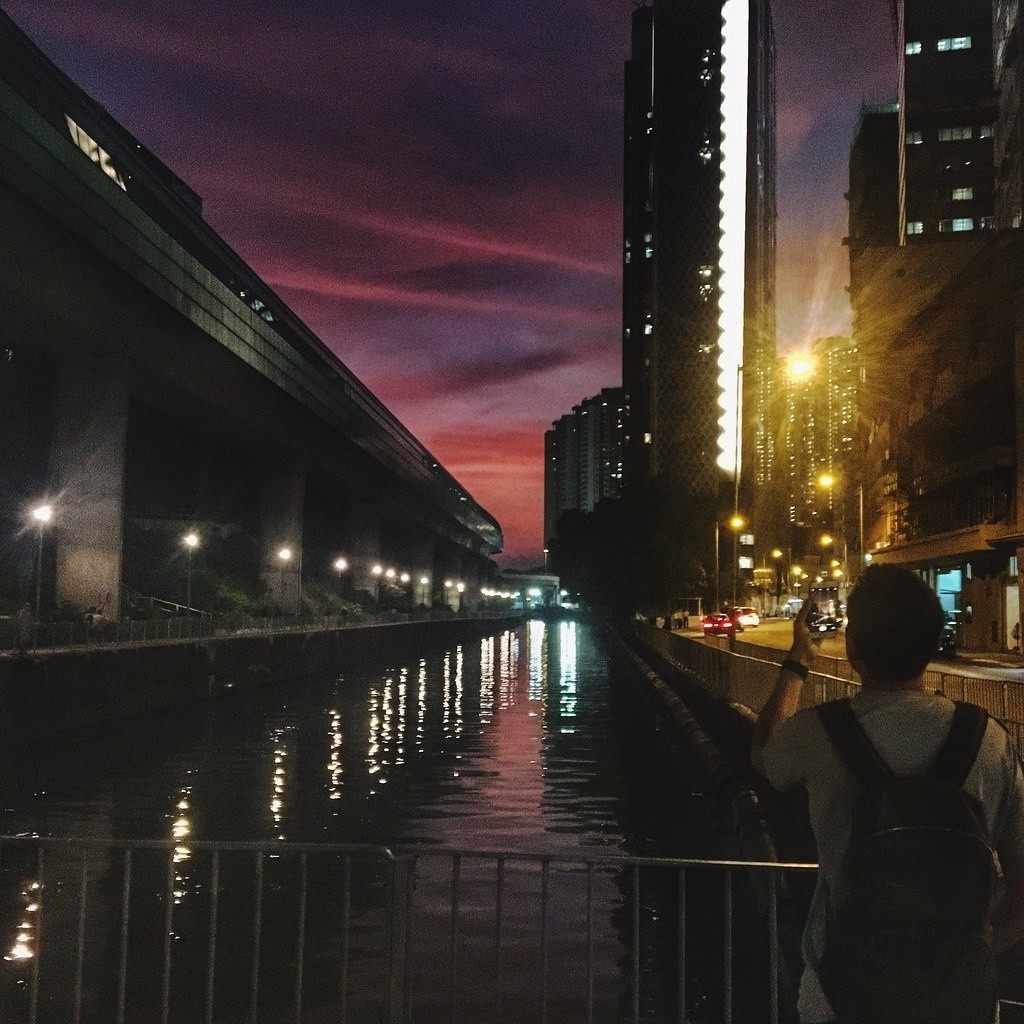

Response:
(702, 611), (735, 637)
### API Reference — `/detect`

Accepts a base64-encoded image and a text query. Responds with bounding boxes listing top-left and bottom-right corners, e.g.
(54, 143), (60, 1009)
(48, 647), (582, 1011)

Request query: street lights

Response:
(179, 526), (202, 615)
(25, 498), (59, 646)
(765, 545), (805, 620)
(331, 556), (522, 615)
(815, 471), (865, 575)
(540, 548), (551, 574)
(278, 542), (295, 620)
(712, 516), (745, 614)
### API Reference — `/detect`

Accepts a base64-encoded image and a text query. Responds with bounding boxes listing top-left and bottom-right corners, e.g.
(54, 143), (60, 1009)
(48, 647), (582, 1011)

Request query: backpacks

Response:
(803, 697), (1008, 1023)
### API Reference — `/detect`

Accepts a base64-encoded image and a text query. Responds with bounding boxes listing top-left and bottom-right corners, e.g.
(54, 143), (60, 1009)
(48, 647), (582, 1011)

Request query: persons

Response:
(751, 562), (1023, 1023)
(671, 606), (690, 630)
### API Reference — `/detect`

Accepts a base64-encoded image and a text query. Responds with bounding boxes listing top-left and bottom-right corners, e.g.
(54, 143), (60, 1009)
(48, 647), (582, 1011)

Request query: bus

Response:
(809, 579), (840, 609)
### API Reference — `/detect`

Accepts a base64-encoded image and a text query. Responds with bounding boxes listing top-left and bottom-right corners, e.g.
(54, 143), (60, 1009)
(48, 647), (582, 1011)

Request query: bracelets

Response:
(781, 661), (807, 681)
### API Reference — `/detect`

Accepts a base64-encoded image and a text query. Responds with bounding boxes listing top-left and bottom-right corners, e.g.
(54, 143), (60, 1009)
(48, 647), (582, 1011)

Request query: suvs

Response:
(731, 606), (760, 627)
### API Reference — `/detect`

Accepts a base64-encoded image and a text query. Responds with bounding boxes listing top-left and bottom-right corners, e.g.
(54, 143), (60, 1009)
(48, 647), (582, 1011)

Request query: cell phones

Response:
(809, 580), (840, 639)
